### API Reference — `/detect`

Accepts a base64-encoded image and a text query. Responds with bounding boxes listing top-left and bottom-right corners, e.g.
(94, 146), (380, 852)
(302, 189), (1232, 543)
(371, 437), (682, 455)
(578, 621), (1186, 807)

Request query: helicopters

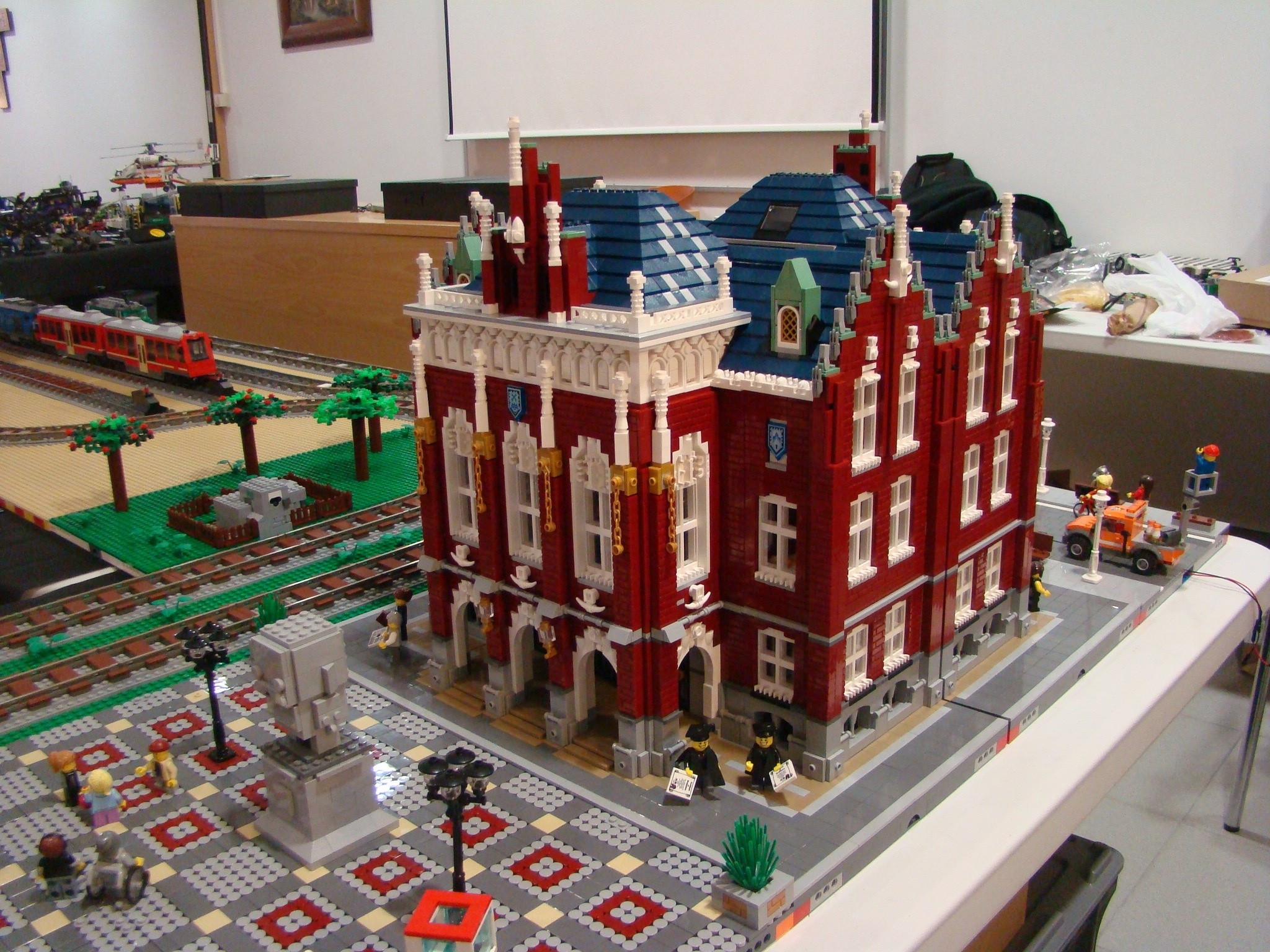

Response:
(97, 140), (223, 192)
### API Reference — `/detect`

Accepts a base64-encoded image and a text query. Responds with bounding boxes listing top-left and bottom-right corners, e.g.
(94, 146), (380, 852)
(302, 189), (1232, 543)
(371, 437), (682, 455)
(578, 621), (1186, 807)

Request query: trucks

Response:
(1060, 500), (1187, 577)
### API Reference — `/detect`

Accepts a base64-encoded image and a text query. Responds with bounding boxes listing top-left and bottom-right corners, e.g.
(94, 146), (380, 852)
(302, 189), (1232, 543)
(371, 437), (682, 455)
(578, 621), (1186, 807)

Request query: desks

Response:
(170, 201), (473, 377)
(1043, 303), (1270, 375)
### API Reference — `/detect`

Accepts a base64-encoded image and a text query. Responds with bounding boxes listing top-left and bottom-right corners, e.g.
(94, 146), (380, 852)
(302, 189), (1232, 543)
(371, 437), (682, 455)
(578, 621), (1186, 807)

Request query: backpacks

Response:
(899, 152), (1073, 270)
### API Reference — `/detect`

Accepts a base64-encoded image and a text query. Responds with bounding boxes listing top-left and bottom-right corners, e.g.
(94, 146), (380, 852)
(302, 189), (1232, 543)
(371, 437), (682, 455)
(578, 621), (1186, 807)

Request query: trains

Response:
(82, 295), (154, 324)
(0, 295), (234, 397)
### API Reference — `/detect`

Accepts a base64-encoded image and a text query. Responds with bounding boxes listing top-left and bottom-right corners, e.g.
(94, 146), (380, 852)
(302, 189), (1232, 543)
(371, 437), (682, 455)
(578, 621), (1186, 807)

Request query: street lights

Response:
(173, 621), (237, 764)
(418, 745), (495, 926)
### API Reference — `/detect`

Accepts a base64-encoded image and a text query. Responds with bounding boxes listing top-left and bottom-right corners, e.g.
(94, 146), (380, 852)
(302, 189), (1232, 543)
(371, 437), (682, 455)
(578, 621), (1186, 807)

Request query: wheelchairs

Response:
(84, 857), (151, 906)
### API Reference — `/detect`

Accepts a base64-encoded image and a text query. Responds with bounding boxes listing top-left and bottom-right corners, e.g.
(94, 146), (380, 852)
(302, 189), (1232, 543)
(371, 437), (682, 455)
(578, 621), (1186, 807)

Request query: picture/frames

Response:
(276, 0), (375, 49)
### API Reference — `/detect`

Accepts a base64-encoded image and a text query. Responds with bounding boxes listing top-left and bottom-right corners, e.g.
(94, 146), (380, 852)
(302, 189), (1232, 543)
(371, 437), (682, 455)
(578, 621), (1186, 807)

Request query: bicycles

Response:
(1073, 492), (1097, 518)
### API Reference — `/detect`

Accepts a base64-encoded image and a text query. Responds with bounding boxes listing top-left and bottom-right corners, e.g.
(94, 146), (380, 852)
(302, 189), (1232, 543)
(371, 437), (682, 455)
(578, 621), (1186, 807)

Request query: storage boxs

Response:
(380, 174), (604, 223)
(960, 832), (1125, 952)
(1218, 266), (1270, 329)
(176, 177), (359, 219)
